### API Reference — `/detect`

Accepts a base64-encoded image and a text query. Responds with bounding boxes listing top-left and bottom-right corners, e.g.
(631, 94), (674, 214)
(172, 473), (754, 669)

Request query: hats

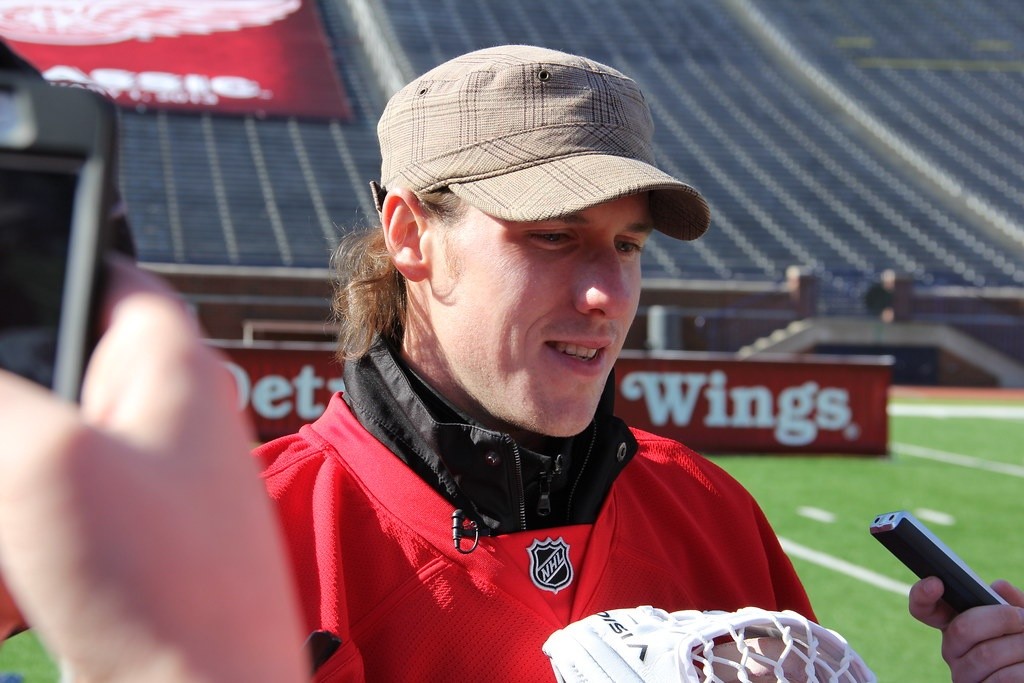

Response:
(370, 44), (711, 243)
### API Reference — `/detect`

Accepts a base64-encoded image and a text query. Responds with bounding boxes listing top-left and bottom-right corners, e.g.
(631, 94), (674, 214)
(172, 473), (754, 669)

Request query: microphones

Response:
(452, 509), (465, 549)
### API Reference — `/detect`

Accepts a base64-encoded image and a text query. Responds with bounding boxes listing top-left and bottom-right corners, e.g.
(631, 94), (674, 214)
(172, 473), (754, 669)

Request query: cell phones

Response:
(0, 72), (120, 404)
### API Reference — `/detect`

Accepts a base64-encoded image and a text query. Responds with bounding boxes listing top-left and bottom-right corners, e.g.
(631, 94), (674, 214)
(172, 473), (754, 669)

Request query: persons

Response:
(1, 253), (309, 683)
(909, 577), (1024, 683)
(249, 43), (878, 683)
